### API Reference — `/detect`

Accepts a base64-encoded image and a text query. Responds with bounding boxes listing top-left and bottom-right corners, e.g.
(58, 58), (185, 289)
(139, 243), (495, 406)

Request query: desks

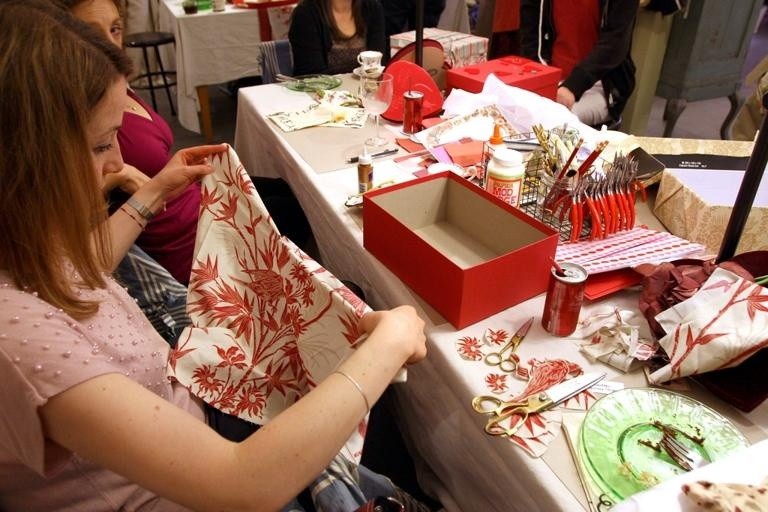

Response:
(149, 0), (298, 135)
(231, 64), (768, 512)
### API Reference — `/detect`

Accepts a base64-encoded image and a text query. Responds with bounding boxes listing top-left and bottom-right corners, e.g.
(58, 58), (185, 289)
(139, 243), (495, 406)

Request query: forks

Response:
(657, 428), (710, 473)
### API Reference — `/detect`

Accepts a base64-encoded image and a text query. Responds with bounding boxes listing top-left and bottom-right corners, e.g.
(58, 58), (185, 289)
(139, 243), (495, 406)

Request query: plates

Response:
(351, 67), (388, 79)
(284, 75), (342, 93)
(581, 385), (753, 500)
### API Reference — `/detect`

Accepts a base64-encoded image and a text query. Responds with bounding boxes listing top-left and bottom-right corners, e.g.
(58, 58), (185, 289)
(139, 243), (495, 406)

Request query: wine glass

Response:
(360, 72), (394, 148)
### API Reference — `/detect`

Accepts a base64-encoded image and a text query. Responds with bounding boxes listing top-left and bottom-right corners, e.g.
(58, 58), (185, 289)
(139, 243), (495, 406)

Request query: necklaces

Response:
(128, 102), (138, 111)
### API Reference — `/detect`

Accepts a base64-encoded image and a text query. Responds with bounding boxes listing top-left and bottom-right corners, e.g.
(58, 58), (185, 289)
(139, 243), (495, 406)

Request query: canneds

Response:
(403, 90), (424, 135)
(542, 261), (588, 337)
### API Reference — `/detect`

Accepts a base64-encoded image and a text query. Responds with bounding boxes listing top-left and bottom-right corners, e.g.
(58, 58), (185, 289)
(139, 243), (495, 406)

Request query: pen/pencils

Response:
(532, 123), (609, 211)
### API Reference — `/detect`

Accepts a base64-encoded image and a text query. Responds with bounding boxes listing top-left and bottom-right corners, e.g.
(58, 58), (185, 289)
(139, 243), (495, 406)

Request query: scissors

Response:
(472, 371), (606, 434)
(486, 316), (534, 372)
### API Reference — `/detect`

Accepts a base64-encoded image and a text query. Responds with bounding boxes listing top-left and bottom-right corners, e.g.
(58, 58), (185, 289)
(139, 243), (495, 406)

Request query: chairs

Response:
(120, 28), (182, 116)
(261, 38), (297, 83)
(216, 0), (297, 127)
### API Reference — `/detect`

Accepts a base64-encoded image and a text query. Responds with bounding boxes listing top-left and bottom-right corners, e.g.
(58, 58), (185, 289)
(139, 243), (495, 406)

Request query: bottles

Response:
(485, 147), (525, 211)
(356, 144), (373, 193)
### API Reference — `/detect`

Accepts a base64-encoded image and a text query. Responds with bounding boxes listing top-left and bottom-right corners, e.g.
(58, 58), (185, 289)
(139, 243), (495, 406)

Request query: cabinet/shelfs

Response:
(653, 1), (765, 140)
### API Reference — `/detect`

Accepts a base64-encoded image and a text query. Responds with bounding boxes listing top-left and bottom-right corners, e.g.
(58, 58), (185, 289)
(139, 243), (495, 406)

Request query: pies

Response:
(682, 480), (768, 512)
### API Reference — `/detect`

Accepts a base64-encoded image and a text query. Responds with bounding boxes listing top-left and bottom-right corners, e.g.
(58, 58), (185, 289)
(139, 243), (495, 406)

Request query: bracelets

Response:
(334, 370), (372, 414)
(126, 197), (154, 222)
(119, 204), (147, 231)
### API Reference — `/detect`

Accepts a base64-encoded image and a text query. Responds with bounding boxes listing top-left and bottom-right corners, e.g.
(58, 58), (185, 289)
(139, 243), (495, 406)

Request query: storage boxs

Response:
(360, 170), (560, 331)
(654, 167), (767, 254)
(388, 25), (489, 66)
(445, 55), (562, 104)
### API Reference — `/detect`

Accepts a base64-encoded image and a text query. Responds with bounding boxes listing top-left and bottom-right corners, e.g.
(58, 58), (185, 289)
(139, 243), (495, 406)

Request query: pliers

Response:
(552, 150), (646, 242)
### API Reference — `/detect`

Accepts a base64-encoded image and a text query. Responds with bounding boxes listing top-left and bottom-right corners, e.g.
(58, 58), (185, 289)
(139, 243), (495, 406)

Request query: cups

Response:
(358, 49), (383, 72)
(178, 0), (247, 16)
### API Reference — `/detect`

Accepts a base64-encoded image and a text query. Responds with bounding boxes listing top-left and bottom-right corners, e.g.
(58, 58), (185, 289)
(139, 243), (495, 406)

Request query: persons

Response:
(0, 2), (428, 511)
(493, 1), (635, 126)
(287, 0), (390, 73)
(53, 1), (317, 282)
